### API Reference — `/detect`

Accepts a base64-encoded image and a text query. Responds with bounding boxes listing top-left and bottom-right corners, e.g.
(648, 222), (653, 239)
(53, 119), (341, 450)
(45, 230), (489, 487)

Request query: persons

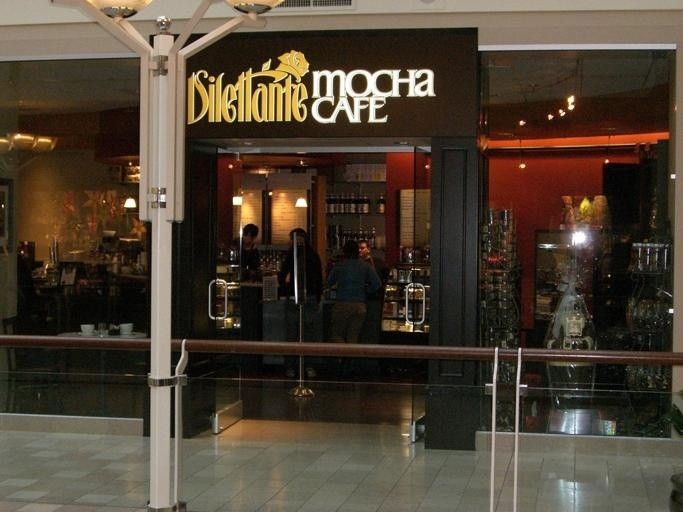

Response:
(228, 224), (382, 379)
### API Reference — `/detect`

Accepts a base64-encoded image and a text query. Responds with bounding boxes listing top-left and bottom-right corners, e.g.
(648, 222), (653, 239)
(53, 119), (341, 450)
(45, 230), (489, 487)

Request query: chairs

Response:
(3, 312), (67, 414)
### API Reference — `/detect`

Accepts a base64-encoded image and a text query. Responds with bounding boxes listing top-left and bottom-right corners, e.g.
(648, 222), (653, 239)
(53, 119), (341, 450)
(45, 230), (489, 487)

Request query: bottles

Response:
(325, 191), (385, 248)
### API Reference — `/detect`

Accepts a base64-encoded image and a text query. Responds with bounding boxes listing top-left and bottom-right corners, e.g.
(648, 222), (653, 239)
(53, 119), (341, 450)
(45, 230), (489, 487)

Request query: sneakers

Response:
(285, 367), (316, 378)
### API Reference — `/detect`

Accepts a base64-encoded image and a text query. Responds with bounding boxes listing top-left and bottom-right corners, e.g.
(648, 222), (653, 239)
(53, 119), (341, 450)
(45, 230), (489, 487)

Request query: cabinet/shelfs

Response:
(382, 282), (434, 334)
(626, 267), (673, 422)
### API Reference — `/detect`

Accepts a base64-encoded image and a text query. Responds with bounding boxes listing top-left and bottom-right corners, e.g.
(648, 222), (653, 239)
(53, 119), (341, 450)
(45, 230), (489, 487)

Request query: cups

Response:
(80, 324), (96, 332)
(118, 323), (133, 332)
(97, 323), (107, 337)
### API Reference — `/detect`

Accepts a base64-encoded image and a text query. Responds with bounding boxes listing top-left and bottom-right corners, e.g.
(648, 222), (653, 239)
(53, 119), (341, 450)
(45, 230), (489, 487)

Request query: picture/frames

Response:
(0, 177), (17, 255)
